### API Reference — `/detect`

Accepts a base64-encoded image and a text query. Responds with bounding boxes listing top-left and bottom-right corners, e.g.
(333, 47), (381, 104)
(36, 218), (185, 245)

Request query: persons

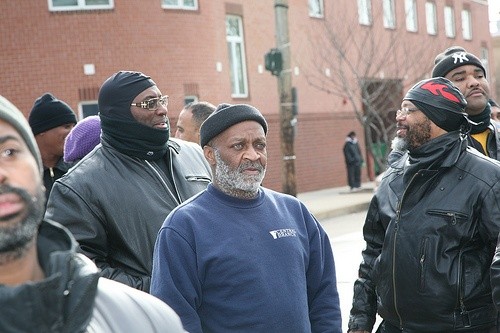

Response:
(151, 103), (343, 333)
(343, 132), (363, 192)
(29, 91), (102, 218)
(175, 101), (216, 148)
(0, 93), (184, 333)
(348, 77), (500, 333)
(42, 69), (212, 293)
(431, 46), (500, 161)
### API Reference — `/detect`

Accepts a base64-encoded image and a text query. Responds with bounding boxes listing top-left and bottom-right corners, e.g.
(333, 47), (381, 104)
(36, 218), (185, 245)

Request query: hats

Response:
(99, 72), (171, 159)
(403, 76), (468, 117)
(64, 115), (101, 164)
(0, 96), (43, 176)
(28, 92), (78, 135)
(431, 47), (486, 78)
(199, 103), (268, 147)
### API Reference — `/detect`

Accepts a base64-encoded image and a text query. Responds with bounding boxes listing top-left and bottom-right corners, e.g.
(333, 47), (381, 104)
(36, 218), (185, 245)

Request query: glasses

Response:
(396, 108), (418, 118)
(131, 95), (168, 111)
